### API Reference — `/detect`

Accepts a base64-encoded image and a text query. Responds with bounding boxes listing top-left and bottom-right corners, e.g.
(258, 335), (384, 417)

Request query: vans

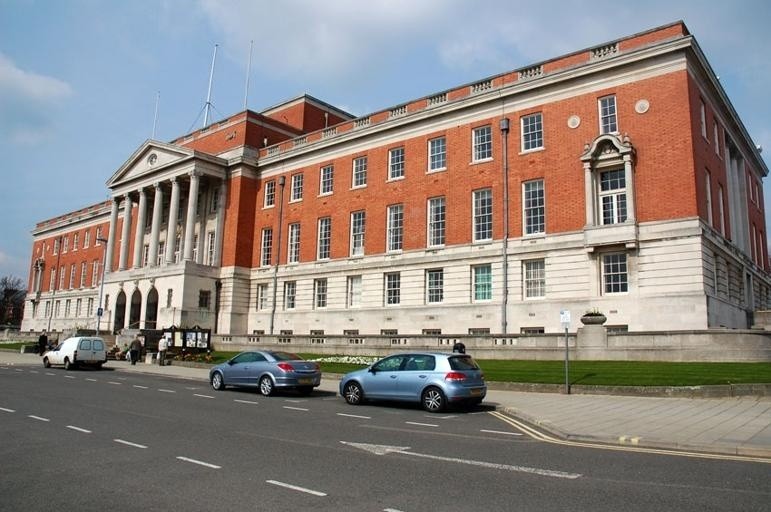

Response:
(43, 337), (108, 370)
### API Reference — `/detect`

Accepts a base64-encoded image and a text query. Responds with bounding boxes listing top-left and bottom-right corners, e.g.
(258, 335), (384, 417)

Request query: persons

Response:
(130, 334), (142, 365)
(39, 328), (48, 356)
(116, 343), (129, 360)
(159, 335), (169, 365)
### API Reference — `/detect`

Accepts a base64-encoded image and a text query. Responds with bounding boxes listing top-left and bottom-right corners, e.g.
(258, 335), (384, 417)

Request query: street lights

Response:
(96, 237), (108, 336)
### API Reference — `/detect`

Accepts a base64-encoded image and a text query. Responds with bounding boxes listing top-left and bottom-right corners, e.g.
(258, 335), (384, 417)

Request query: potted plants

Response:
(581, 308), (607, 325)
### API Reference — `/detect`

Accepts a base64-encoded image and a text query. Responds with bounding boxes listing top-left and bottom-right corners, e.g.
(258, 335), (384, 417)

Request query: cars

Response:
(209, 349), (321, 396)
(340, 352), (487, 413)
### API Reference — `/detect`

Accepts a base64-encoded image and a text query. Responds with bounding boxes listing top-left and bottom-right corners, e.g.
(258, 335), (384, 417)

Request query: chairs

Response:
(424, 358), (435, 369)
(406, 360), (418, 370)
